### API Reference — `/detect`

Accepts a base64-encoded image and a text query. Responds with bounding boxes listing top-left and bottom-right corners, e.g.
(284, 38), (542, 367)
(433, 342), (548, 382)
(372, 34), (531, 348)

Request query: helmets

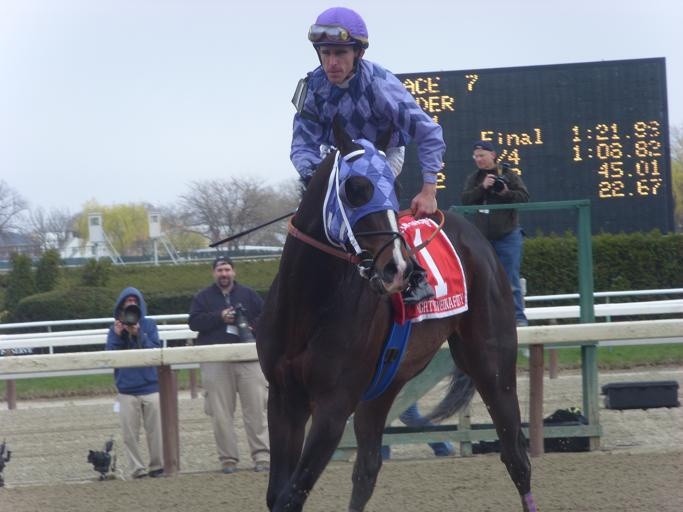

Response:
(307, 5), (370, 50)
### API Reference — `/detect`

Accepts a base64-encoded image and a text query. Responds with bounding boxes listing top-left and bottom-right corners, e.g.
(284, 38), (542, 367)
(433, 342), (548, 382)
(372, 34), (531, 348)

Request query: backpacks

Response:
(542, 405), (591, 453)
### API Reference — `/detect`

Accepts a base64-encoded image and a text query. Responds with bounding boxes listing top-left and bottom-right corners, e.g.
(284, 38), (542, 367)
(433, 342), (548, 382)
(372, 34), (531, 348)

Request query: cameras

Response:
(493, 163), (511, 192)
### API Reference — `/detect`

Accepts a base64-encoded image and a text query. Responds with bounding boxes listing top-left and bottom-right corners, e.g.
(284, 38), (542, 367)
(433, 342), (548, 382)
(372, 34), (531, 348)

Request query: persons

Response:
(103, 286), (166, 479)
(458, 140), (531, 328)
(288, 5), (447, 221)
(188, 256), (273, 475)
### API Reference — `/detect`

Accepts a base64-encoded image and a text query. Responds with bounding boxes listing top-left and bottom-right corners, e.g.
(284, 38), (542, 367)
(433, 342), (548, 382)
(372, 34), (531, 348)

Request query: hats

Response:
(212, 256), (235, 272)
(472, 140), (498, 154)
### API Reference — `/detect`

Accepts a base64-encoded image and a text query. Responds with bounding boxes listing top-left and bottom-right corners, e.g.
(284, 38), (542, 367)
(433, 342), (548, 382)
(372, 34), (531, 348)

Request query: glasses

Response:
(306, 23), (353, 43)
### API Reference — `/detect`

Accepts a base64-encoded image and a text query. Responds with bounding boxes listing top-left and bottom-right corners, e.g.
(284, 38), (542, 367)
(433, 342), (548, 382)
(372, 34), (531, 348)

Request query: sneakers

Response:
(394, 268), (437, 303)
(222, 462), (239, 475)
(253, 460), (271, 473)
(134, 467), (169, 480)
(515, 319), (529, 329)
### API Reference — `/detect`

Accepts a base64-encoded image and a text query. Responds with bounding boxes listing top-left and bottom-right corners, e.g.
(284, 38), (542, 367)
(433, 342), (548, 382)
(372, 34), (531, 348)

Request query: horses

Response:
(254, 112), (536, 511)
